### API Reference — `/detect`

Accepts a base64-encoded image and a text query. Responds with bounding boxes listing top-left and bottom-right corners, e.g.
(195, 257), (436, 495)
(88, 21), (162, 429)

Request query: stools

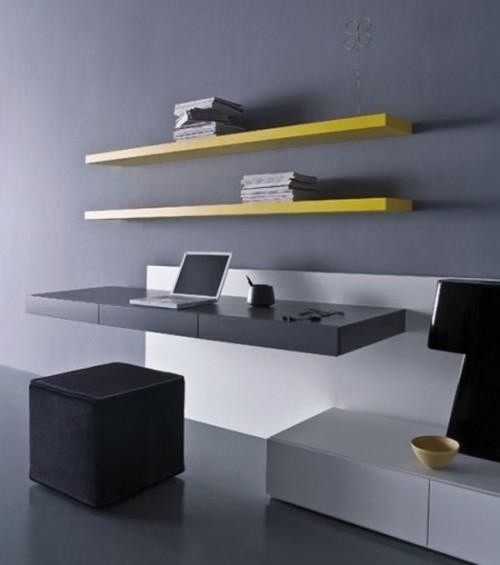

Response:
(28, 360), (186, 509)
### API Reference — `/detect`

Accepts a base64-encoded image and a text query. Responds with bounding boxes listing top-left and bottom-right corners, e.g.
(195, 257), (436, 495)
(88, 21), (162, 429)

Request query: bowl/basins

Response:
(408, 434), (460, 470)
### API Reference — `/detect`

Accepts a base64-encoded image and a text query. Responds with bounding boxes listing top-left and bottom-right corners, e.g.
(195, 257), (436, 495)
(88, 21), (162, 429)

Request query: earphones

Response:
(288, 316), (296, 322)
(282, 315), (288, 323)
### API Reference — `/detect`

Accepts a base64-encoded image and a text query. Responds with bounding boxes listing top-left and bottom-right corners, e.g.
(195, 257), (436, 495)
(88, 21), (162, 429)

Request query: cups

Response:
(247, 284), (275, 307)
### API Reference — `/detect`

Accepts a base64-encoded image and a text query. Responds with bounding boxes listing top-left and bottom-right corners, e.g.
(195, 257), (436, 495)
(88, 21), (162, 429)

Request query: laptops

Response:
(128, 250), (233, 310)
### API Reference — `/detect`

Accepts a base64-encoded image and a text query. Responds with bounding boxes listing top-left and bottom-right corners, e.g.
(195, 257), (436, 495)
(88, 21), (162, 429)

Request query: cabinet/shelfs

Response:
(265, 406), (500, 565)
(82, 112), (414, 221)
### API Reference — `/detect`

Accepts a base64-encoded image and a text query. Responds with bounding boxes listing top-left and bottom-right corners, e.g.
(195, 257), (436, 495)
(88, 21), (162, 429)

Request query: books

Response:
(173, 96), (245, 141)
(240, 172), (318, 203)
